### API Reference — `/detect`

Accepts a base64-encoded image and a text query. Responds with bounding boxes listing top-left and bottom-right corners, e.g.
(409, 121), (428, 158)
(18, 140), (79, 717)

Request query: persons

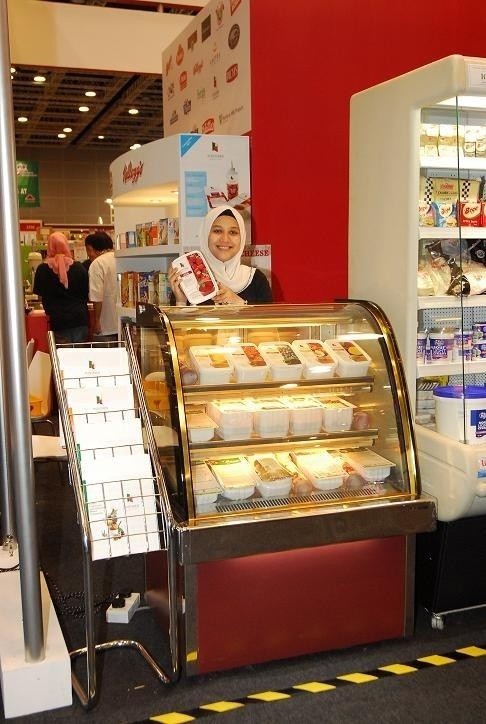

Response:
(33, 231), (88, 347)
(166, 205), (271, 307)
(85, 234), (118, 348)
(81, 235), (95, 271)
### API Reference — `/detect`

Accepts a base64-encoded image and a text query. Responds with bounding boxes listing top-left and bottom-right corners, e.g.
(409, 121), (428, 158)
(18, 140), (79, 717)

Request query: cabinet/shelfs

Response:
(108, 185), (190, 315)
(137, 294), (438, 683)
(42, 322), (182, 709)
(347, 109), (486, 523)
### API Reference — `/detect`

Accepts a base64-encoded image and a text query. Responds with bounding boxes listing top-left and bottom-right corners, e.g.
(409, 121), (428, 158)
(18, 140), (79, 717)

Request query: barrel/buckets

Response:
(433, 386), (486, 477)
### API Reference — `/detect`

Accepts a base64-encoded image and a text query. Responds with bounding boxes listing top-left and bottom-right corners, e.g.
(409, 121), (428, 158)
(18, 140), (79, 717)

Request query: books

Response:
(54, 347), (162, 561)
